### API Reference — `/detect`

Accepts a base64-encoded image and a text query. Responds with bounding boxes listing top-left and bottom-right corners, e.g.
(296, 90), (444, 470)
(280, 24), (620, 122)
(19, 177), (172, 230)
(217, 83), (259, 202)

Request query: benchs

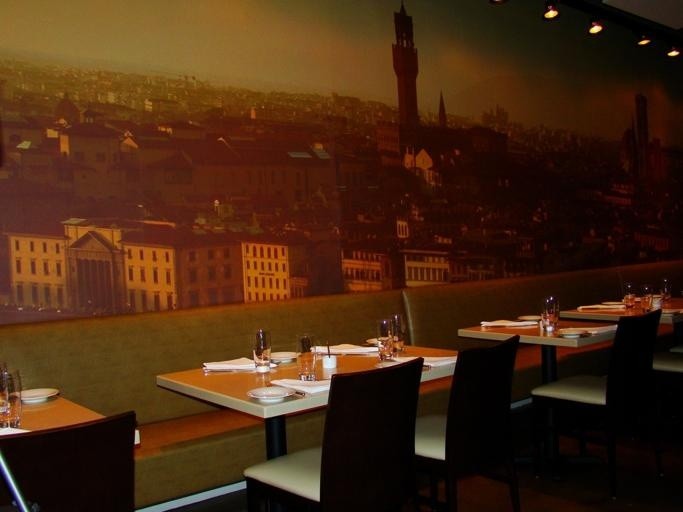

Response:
(402, 265), (624, 406)
(1, 289), (412, 512)
(623, 259), (682, 299)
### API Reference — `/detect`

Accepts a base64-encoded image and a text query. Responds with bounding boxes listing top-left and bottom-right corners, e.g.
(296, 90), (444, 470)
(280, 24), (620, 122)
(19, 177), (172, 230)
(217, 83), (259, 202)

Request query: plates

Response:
(271, 351), (300, 362)
(555, 329), (587, 338)
(247, 387), (296, 402)
(366, 336), (398, 346)
(602, 301), (623, 305)
(517, 315), (541, 321)
(16, 388), (60, 403)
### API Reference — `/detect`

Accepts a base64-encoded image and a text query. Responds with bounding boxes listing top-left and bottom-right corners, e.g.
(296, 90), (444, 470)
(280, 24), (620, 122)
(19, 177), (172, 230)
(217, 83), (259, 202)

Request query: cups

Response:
(623, 278), (671, 309)
(252, 329), (273, 372)
(376, 319), (394, 362)
(392, 313), (409, 352)
(295, 333), (316, 382)
(0, 368), (22, 429)
(541, 295), (562, 331)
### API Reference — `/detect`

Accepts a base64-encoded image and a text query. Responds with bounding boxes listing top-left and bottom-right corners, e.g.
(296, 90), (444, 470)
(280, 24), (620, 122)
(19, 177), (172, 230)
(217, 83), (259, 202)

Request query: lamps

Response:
(543, 0), (682, 57)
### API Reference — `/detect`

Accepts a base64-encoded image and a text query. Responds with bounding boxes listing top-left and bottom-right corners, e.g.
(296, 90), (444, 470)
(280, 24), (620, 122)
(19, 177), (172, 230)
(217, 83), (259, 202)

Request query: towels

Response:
(576, 304), (624, 310)
